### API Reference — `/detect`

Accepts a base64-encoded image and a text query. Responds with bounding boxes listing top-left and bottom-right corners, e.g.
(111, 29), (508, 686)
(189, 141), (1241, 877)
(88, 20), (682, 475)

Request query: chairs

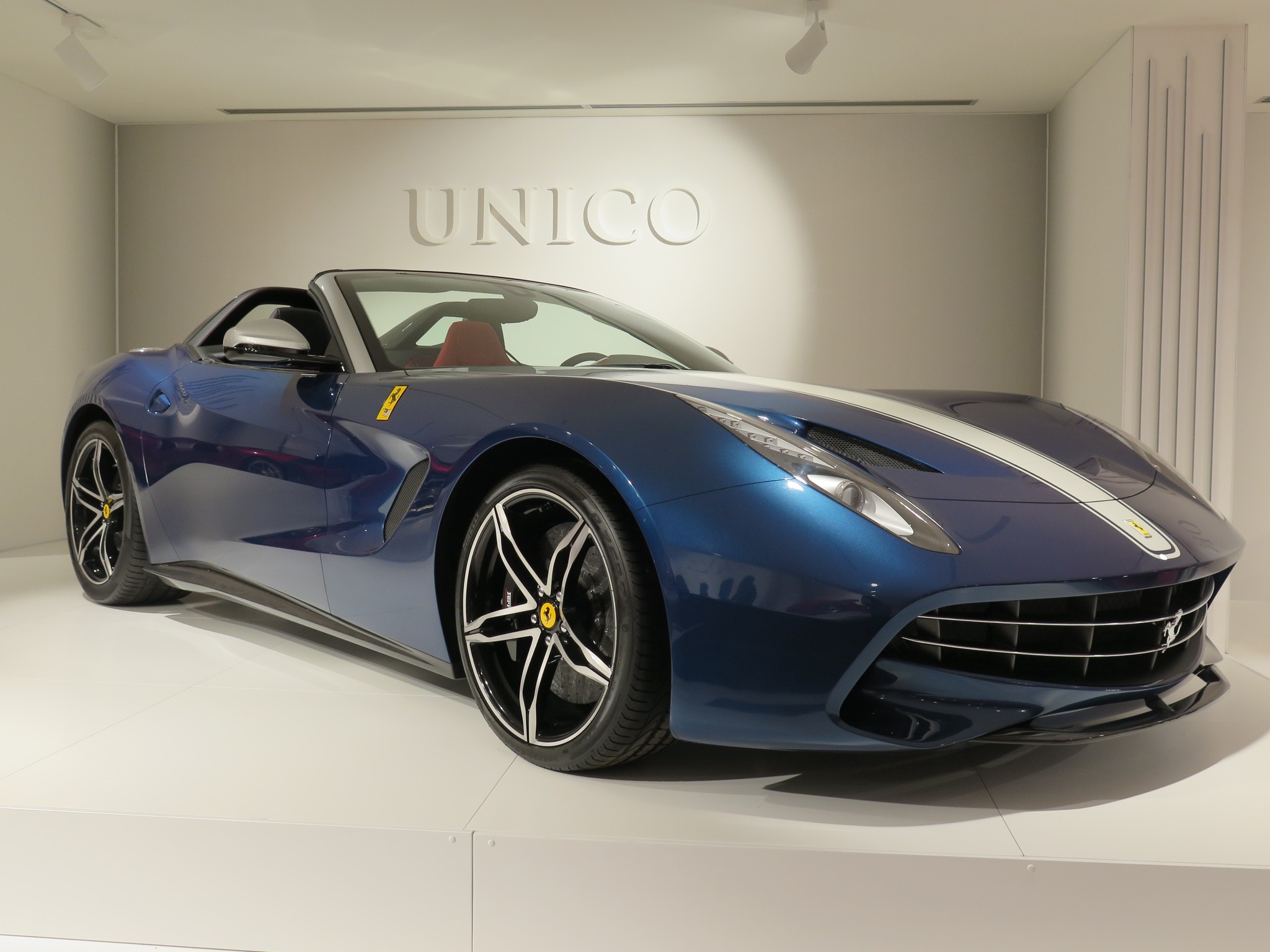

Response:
(431, 319), (516, 369)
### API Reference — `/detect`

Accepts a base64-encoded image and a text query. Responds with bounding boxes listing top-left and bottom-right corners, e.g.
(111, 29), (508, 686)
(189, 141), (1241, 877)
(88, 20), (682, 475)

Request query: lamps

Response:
(52, 31), (110, 93)
(784, 18), (828, 75)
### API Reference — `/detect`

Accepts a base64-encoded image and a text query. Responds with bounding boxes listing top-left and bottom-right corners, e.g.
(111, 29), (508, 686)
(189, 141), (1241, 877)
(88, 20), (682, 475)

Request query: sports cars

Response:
(59, 266), (1241, 776)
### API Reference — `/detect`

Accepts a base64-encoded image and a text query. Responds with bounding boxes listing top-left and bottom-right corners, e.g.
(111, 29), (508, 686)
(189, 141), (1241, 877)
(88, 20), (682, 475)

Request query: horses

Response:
(1161, 608), (1184, 654)
